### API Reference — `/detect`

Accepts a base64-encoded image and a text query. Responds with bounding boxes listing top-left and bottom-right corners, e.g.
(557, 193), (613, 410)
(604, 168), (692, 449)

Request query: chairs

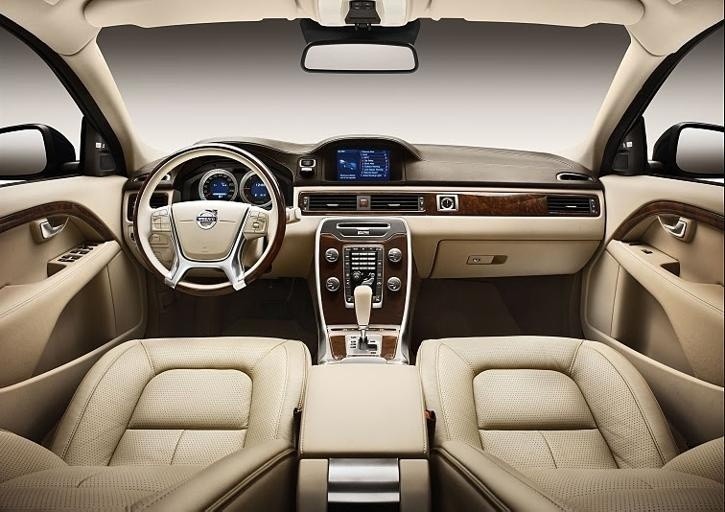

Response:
(1, 337), (311, 510)
(415, 336), (724, 510)
